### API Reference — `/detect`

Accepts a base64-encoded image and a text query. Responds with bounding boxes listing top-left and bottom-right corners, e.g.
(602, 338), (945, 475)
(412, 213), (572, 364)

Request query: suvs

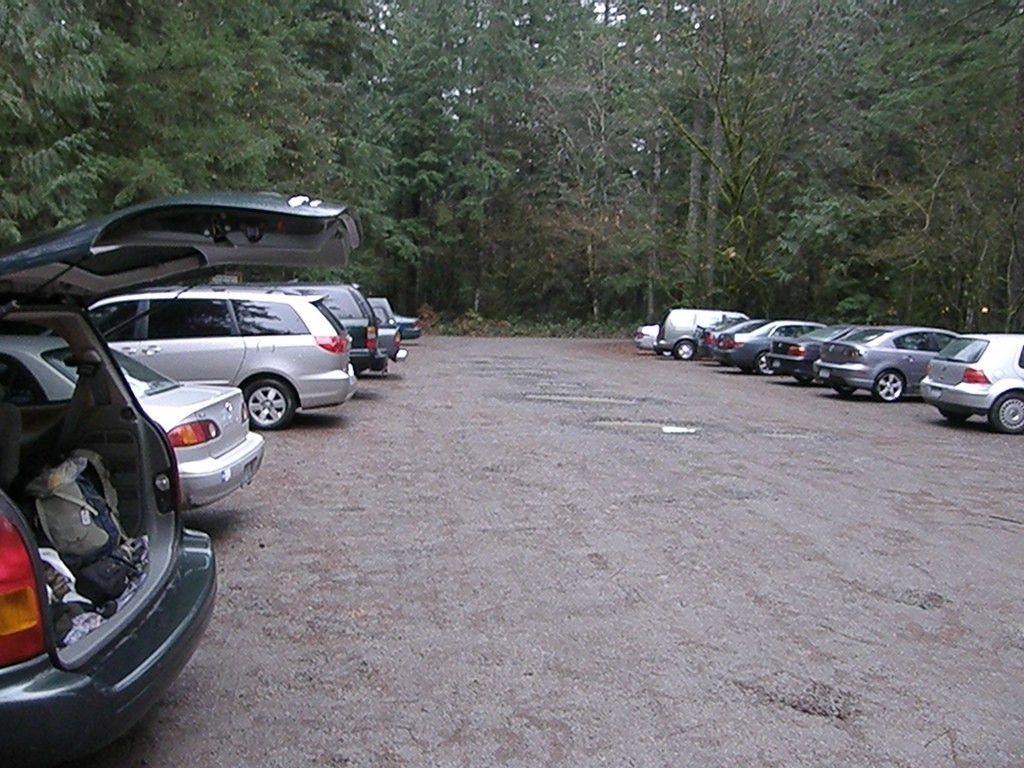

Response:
(654, 309), (750, 361)
(0, 192), (361, 768)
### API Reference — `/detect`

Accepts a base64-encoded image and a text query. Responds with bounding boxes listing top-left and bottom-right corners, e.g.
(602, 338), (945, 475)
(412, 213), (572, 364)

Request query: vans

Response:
(44, 279), (408, 431)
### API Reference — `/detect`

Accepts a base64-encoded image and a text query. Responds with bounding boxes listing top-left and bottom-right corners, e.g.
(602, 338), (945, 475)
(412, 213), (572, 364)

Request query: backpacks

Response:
(28, 456), (145, 605)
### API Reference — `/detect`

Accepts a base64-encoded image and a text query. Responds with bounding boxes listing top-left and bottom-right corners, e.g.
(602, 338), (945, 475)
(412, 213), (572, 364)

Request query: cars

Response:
(921, 333), (1024, 433)
(697, 315), (828, 376)
(813, 325), (961, 403)
(394, 314), (422, 343)
(765, 324), (852, 387)
(635, 324), (671, 357)
(0, 335), (265, 510)
